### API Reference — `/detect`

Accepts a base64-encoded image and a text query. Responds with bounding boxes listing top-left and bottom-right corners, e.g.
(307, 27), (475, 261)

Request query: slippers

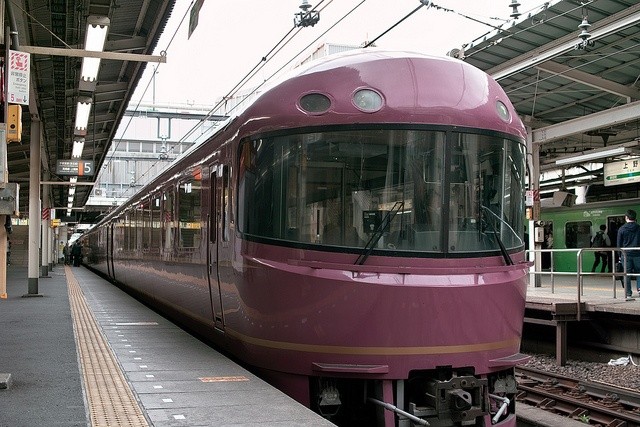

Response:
(615, 261), (624, 289)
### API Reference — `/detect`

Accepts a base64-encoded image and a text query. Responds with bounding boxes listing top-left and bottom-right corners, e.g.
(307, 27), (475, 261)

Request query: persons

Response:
(590, 225), (611, 273)
(542, 222), (554, 269)
(57, 239), (83, 267)
(617, 210), (640, 298)
(607, 222), (624, 272)
